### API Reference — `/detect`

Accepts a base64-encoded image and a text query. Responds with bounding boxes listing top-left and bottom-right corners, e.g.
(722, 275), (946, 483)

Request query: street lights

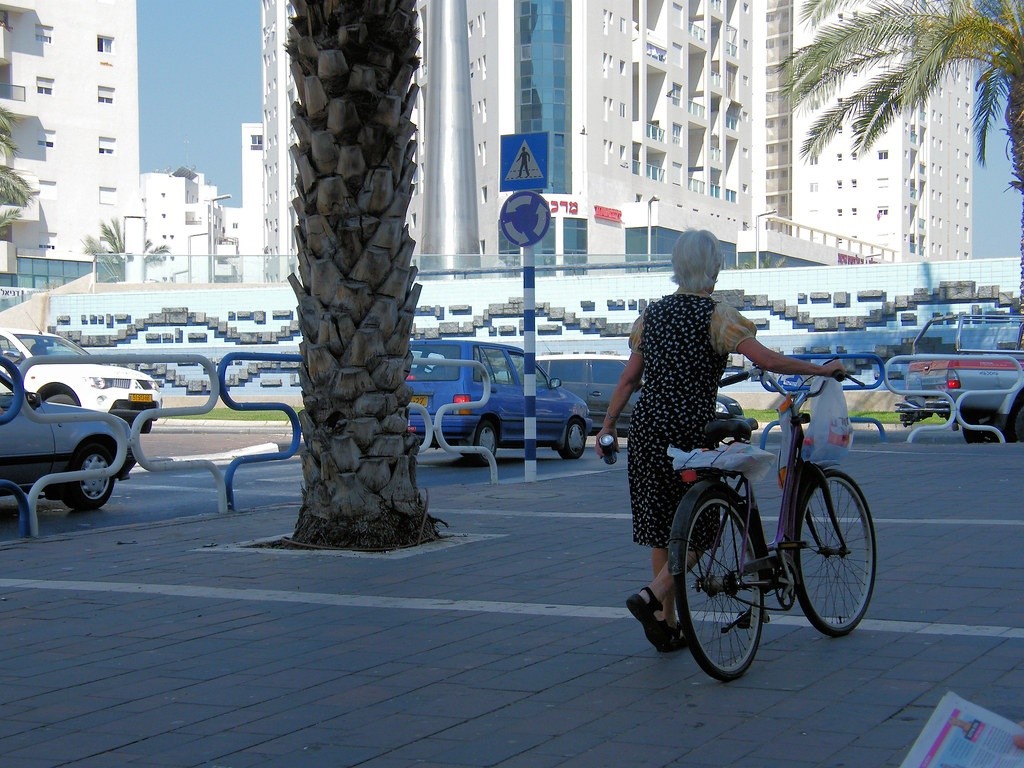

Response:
(209, 194), (232, 283)
(646, 196), (661, 262)
(755, 210), (778, 270)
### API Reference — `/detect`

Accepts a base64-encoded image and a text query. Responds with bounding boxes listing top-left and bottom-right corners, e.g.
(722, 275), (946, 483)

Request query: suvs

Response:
(897, 314), (1024, 444)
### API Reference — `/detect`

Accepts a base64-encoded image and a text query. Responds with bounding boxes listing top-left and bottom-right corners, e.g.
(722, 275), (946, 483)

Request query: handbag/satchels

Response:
(801, 375), (851, 468)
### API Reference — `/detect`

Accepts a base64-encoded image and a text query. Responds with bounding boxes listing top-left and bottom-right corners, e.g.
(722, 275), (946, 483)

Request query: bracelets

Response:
(606, 407), (619, 421)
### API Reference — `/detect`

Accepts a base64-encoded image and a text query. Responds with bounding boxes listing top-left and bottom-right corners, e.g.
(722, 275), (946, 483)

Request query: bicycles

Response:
(667, 356), (879, 682)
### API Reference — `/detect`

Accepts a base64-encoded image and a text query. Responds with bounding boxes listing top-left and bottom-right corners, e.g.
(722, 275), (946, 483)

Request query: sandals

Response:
(625, 586), (673, 650)
(656, 620), (688, 652)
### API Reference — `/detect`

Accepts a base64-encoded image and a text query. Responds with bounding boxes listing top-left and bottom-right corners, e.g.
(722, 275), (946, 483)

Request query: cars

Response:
(511, 351), (746, 439)
(0, 325), (163, 510)
(402, 340), (595, 468)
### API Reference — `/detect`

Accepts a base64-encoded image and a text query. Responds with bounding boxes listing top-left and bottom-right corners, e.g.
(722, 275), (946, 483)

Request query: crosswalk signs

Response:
(500, 130), (548, 191)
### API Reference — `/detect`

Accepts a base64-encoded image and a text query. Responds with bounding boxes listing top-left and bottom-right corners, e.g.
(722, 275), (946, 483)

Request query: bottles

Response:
(802, 438), (814, 462)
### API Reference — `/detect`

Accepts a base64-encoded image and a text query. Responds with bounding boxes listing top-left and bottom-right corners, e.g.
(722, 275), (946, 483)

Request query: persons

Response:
(1013, 721), (1024, 750)
(594, 228), (847, 652)
